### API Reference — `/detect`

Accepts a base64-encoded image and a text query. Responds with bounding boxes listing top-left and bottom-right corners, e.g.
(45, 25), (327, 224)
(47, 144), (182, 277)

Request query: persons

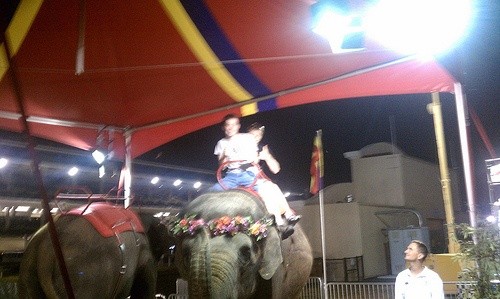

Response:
(205, 113), (302, 240)
(395, 241), (444, 299)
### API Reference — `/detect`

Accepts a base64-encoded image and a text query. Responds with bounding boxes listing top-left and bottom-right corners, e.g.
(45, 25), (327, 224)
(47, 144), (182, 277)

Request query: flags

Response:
(309, 136), (325, 195)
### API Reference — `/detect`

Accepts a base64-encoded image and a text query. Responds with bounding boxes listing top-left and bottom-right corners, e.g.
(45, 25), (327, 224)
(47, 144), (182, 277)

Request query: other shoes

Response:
(277, 224), (295, 240)
(288, 214), (302, 225)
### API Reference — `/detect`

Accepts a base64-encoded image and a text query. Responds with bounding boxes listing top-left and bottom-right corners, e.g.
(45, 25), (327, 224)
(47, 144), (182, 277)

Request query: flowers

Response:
(169, 213), (273, 242)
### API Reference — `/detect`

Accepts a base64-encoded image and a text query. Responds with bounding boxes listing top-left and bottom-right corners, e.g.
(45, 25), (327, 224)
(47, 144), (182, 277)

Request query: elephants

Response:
(158, 189), (314, 298)
(18, 205), (177, 298)
